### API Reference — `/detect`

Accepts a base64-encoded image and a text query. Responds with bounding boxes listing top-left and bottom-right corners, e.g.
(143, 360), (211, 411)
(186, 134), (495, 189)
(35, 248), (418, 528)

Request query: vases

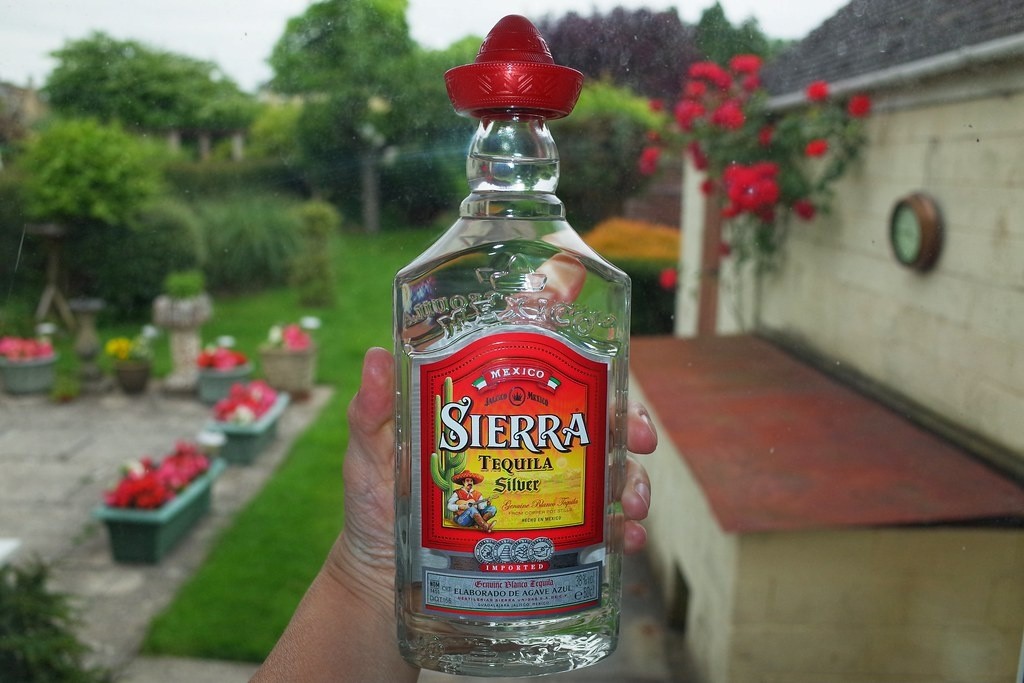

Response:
(114, 361), (150, 394)
(0, 355), (58, 394)
(96, 457), (228, 562)
(207, 391), (291, 463)
(258, 342), (317, 399)
(201, 367), (254, 406)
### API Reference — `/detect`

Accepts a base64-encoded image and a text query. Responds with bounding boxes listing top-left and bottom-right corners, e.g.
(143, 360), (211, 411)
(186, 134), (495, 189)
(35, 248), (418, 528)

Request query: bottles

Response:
(392, 12), (632, 678)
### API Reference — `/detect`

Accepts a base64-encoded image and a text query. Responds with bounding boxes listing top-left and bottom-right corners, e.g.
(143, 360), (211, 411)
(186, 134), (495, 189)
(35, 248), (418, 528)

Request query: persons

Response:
(246, 252), (659, 683)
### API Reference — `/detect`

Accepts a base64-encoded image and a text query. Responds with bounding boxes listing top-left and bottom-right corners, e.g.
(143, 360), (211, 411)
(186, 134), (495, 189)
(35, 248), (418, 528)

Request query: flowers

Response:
(216, 380), (278, 424)
(104, 324), (159, 367)
(266, 314), (322, 349)
(0, 336), (55, 360)
(196, 334), (248, 369)
(100, 434), (210, 507)
